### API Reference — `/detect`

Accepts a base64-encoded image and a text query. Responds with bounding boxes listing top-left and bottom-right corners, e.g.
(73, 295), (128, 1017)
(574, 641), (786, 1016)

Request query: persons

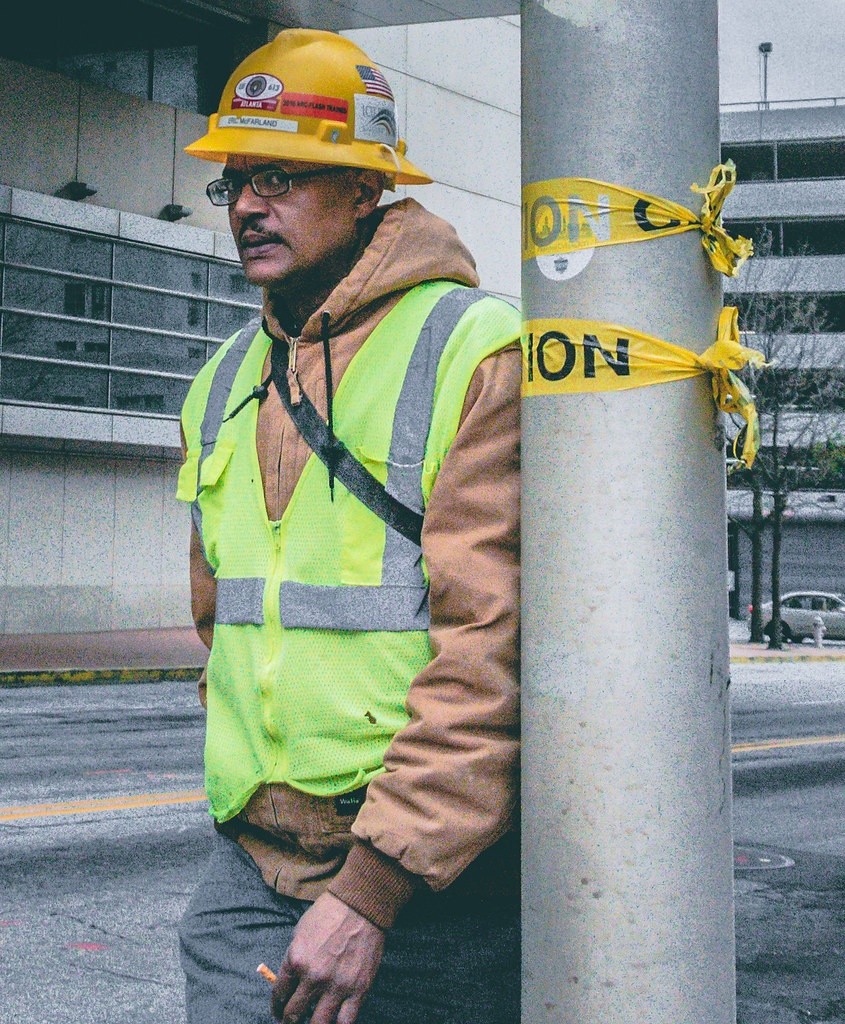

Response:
(179, 29), (523, 1024)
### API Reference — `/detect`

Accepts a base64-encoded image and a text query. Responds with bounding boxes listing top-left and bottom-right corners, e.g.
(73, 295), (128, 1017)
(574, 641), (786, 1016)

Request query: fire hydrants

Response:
(811, 615), (827, 648)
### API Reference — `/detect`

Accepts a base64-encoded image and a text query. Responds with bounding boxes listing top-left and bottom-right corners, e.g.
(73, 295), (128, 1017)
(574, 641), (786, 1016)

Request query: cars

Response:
(746, 591), (845, 643)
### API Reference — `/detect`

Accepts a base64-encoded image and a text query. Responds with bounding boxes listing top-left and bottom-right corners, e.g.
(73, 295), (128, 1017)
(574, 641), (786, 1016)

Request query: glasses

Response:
(205, 165), (355, 206)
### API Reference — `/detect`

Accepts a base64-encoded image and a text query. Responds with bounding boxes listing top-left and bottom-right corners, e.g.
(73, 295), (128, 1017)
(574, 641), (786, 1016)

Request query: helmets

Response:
(183, 29), (434, 184)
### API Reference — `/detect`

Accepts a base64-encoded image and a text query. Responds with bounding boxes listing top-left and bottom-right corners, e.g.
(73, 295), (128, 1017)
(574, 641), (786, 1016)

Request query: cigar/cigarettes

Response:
(257, 963), (277, 984)
